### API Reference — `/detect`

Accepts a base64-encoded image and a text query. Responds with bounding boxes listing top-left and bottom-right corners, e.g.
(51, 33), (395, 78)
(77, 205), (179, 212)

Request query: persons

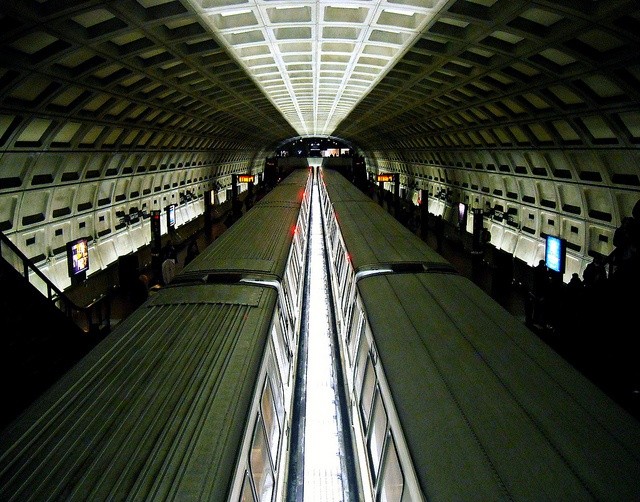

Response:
(186, 241), (200, 264)
(161, 240), (175, 259)
(132, 274), (149, 308)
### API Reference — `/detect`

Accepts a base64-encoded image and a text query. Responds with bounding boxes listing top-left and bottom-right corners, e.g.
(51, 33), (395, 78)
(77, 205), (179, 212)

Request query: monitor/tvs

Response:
(164, 203), (178, 234)
(458, 202), (468, 228)
(417, 189), (428, 211)
(544, 234), (565, 275)
(66, 236), (90, 284)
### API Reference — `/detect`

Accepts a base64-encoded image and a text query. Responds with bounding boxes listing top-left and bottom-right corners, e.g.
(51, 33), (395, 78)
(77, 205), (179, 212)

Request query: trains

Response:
(0, 167), (314, 498)
(317, 166), (637, 502)
